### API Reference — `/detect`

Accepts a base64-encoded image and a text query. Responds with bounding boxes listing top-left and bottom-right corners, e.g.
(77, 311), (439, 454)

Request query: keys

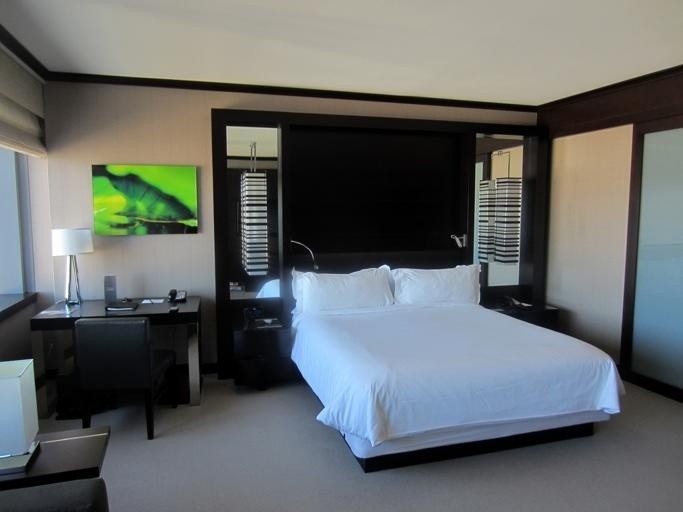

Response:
(121, 297), (131, 303)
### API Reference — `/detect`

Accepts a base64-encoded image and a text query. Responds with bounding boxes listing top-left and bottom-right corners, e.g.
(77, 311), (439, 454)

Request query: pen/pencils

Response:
(150, 299), (154, 305)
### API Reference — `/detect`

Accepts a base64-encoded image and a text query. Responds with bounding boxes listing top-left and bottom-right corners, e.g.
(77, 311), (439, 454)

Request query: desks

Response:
(32, 295), (202, 417)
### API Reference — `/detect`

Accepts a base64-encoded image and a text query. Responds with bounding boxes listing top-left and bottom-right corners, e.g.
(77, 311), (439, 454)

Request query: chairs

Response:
(75, 316), (174, 439)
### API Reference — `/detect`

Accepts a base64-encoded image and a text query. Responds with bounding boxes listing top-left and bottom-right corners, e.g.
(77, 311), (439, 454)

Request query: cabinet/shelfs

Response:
(626, 116), (683, 402)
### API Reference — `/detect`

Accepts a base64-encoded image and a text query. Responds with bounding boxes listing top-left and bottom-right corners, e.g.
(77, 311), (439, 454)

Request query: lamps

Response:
(244, 141), (268, 275)
(53, 228), (92, 305)
(477, 154), (497, 265)
(495, 152), (522, 265)
(240, 143), (254, 269)
(0, 359), (40, 475)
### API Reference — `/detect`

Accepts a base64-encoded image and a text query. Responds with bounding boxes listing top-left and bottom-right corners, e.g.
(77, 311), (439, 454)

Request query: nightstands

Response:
(232, 319), (290, 386)
(489, 302), (558, 331)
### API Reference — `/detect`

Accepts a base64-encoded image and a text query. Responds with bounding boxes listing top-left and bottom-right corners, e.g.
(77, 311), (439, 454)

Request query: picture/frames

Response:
(91, 164), (198, 237)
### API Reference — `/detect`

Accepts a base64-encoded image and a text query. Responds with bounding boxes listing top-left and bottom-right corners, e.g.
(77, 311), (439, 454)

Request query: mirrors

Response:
(227, 125), (283, 301)
(473, 134), (522, 286)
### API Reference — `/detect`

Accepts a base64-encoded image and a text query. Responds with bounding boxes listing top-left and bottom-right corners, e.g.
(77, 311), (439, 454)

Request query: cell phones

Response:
(168, 306), (179, 314)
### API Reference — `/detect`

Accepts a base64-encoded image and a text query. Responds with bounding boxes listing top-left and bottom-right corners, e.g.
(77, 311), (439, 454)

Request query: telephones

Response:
(168, 289), (187, 303)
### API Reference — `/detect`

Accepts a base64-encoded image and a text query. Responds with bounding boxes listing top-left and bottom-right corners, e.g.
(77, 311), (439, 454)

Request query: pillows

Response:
(395, 264), (481, 305)
(295, 265), (392, 312)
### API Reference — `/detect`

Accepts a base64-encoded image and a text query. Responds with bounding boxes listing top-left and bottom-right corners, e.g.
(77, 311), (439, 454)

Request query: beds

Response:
(291, 250), (625, 473)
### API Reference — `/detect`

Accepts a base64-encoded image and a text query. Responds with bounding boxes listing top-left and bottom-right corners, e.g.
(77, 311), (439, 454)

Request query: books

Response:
(104, 301), (139, 311)
(254, 317), (282, 329)
